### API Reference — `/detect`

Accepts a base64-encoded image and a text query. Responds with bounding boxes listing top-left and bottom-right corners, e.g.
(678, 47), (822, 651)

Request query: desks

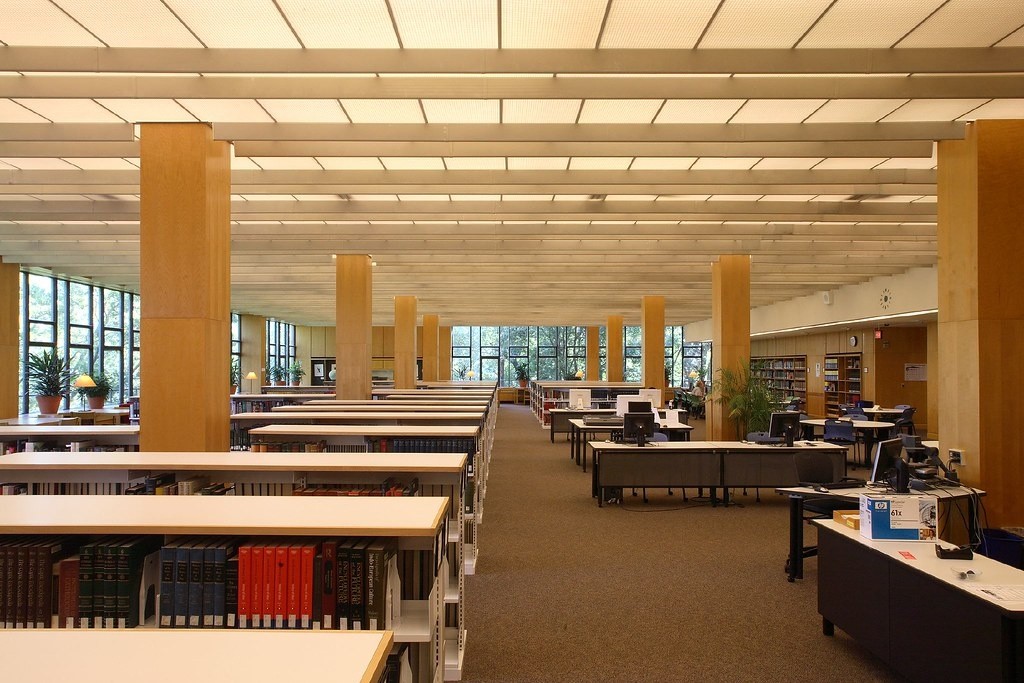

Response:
(550, 407), (1024, 683)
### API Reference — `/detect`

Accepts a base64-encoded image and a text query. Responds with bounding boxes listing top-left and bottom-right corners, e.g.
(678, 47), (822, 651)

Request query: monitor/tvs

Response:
(569, 389), (591, 411)
(871, 438), (910, 494)
(769, 413), (801, 447)
(617, 389), (661, 447)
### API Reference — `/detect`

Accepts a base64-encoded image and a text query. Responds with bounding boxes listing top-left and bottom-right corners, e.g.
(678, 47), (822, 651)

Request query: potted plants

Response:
(272, 366), (286, 386)
(265, 362), (271, 386)
(287, 362), (306, 386)
(665, 365), (672, 387)
(18, 348), (78, 414)
(514, 363), (532, 387)
(79, 368), (114, 409)
(230, 357), (245, 394)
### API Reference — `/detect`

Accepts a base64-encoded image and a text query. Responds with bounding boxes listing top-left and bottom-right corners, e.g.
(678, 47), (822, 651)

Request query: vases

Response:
(565, 378), (582, 380)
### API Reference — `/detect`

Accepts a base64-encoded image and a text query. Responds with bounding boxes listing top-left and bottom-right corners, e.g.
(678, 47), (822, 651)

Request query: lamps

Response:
(71, 376), (97, 414)
(247, 372), (257, 394)
(688, 371), (698, 388)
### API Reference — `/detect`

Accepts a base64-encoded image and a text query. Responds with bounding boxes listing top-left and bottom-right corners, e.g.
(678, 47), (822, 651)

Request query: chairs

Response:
(820, 420), (867, 472)
(846, 407), (865, 415)
(837, 403), (845, 417)
(893, 406), (918, 439)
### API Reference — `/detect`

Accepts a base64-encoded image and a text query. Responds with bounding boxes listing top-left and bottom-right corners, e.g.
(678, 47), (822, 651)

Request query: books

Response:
(0, 391), (475, 683)
(749, 357), (860, 416)
(530, 384), (641, 426)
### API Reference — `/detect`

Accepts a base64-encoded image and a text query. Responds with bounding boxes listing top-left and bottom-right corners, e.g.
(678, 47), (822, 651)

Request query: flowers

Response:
(454, 366), (474, 381)
(567, 371), (583, 378)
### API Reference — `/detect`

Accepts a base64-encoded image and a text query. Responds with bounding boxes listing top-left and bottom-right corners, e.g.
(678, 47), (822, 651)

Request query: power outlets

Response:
(948, 449), (965, 466)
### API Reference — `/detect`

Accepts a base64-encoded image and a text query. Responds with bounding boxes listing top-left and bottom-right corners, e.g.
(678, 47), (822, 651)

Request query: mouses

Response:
(815, 486), (829, 493)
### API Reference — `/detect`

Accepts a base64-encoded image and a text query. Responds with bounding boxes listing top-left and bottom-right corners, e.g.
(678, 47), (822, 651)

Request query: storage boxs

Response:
(860, 492), (939, 543)
(825, 383), (836, 392)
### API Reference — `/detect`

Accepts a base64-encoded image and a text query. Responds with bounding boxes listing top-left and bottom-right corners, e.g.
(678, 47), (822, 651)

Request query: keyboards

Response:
(822, 479), (866, 489)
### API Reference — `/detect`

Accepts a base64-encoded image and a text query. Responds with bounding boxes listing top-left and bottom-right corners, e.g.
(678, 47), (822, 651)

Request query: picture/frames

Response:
(314, 364), (324, 377)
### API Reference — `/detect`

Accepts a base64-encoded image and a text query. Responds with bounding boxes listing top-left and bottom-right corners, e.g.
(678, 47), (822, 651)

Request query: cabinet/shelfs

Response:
(0, 380), (499, 683)
(824, 354), (863, 416)
(750, 356), (807, 411)
(528, 380), (641, 429)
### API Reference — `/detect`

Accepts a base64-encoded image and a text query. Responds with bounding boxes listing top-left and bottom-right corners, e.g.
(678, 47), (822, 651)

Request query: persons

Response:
(691, 380), (705, 415)
(926, 530), (936, 541)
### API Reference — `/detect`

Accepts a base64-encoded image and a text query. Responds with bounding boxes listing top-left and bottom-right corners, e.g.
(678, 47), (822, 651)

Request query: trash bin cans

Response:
(980, 528), (1024, 568)
